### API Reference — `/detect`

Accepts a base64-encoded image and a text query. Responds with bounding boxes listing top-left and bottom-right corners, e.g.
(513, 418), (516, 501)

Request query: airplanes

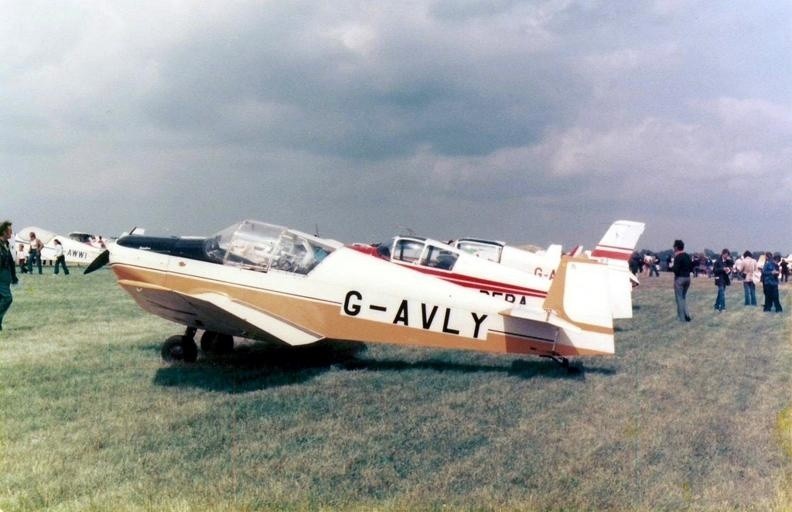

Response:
(83, 219), (646, 361)
(8, 223), (145, 267)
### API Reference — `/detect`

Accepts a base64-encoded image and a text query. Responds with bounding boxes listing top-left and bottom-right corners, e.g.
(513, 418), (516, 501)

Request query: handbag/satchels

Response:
(741, 273), (746, 279)
(753, 271), (762, 285)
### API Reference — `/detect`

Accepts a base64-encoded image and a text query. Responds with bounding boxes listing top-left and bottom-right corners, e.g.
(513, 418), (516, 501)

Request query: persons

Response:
(629, 251), (791, 284)
(17, 243), (30, 274)
(10, 244), (16, 262)
(760, 252), (783, 313)
(0, 221), (20, 333)
(669, 240), (693, 322)
(27, 232), (45, 274)
(739, 250), (758, 305)
(53, 240), (70, 276)
(713, 247), (735, 311)
